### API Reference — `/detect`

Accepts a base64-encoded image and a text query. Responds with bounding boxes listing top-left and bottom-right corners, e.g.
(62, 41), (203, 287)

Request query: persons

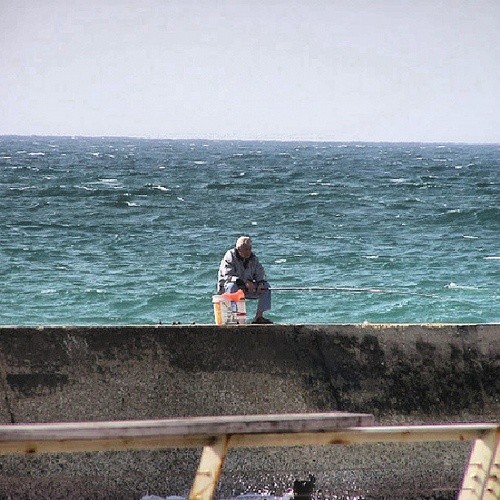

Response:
(217, 236), (273, 324)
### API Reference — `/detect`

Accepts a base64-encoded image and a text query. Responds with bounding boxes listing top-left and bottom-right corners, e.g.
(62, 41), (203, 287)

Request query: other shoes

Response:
(252, 316), (273, 324)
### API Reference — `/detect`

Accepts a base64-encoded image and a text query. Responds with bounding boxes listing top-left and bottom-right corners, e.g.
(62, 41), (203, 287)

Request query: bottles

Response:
(231, 301), (237, 324)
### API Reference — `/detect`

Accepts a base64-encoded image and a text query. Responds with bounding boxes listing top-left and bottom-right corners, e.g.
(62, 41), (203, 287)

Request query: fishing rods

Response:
(245, 286), (444, 296)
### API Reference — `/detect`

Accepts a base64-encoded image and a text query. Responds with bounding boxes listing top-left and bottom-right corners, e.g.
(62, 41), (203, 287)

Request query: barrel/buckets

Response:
(212, 295), (246, 325)
